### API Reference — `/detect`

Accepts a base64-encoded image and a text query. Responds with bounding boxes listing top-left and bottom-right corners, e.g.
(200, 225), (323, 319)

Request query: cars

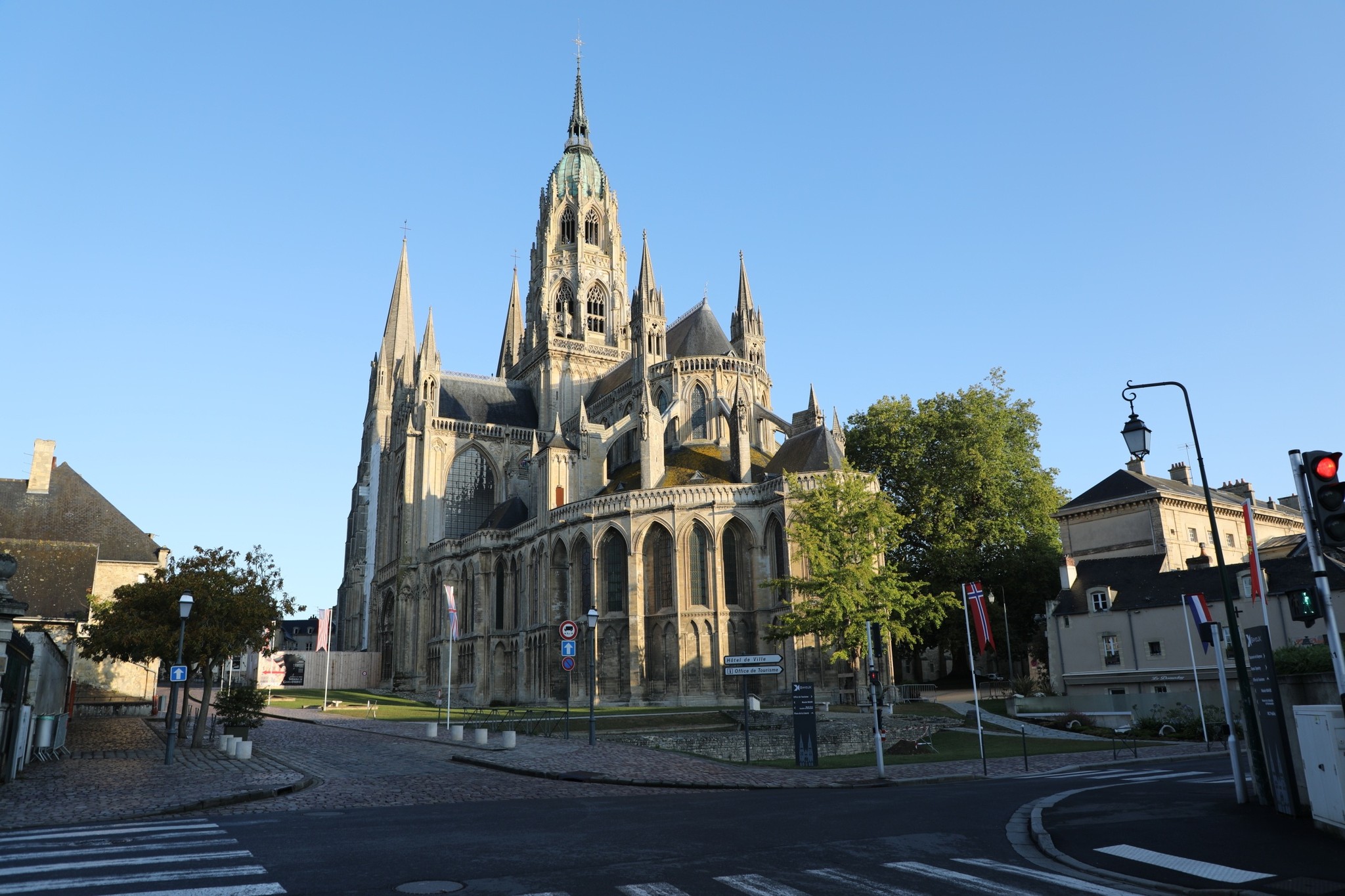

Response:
(986, 674), (1004, 681)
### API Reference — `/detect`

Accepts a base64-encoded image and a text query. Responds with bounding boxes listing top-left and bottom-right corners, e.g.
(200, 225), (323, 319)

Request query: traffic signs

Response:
(724, 654), (784, 665)
(725, 665), (784, 676)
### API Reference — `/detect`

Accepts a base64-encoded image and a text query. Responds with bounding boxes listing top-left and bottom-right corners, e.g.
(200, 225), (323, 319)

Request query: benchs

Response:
(815, 702), (830, 712)
(857, 702), (894, 714)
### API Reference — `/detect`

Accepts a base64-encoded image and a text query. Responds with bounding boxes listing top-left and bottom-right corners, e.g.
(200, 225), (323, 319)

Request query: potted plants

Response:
(212, 677), (270, 740)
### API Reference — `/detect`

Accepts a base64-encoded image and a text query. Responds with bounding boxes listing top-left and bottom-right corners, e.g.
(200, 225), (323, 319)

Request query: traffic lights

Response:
(1303, 450), (1345, 546)
(1286, 585), (1324, 622)
(870, 670), (879, 683)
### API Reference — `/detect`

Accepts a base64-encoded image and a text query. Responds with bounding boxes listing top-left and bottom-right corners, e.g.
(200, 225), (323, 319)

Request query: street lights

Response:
(987, 584), (1015, 694)
(165, 589), (195, 765)
(585, 604), (600, 745)
(1121, 379), (1274, 810)
